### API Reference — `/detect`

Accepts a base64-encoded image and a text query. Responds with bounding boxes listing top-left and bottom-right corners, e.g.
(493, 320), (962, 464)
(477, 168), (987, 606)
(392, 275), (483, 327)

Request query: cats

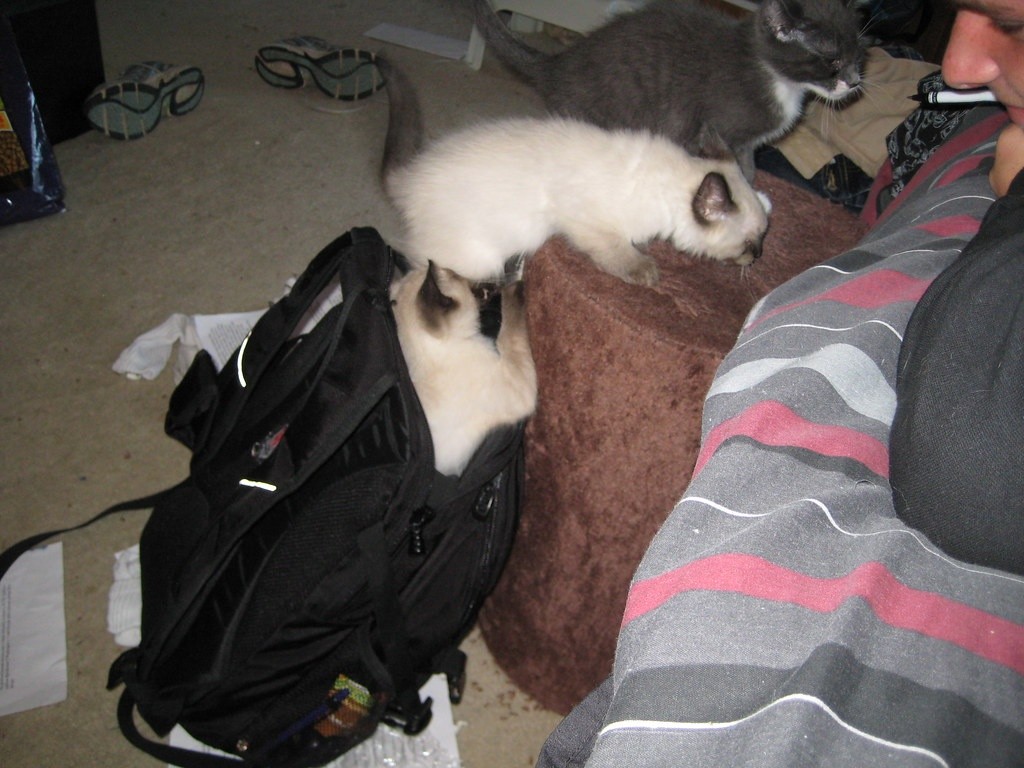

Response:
(373, 0), (866, 478)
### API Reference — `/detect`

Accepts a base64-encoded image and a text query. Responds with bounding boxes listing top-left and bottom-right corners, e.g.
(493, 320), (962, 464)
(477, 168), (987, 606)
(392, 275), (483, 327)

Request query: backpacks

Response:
(106, 223), (528, 768)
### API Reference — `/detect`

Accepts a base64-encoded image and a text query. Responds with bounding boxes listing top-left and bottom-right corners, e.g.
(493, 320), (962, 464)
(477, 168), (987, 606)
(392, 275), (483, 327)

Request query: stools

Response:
(464, 0), (632, 72)
(478, 168), (869, 719)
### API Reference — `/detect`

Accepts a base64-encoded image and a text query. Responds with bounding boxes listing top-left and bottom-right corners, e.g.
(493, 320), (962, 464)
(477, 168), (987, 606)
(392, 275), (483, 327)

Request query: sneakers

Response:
(255, 35), (393, 101)
(84, 55), (203, 141)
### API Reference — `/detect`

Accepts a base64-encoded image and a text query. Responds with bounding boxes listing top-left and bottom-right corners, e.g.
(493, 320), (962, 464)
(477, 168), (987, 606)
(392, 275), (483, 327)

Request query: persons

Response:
(889, 0), (1024, 577)
